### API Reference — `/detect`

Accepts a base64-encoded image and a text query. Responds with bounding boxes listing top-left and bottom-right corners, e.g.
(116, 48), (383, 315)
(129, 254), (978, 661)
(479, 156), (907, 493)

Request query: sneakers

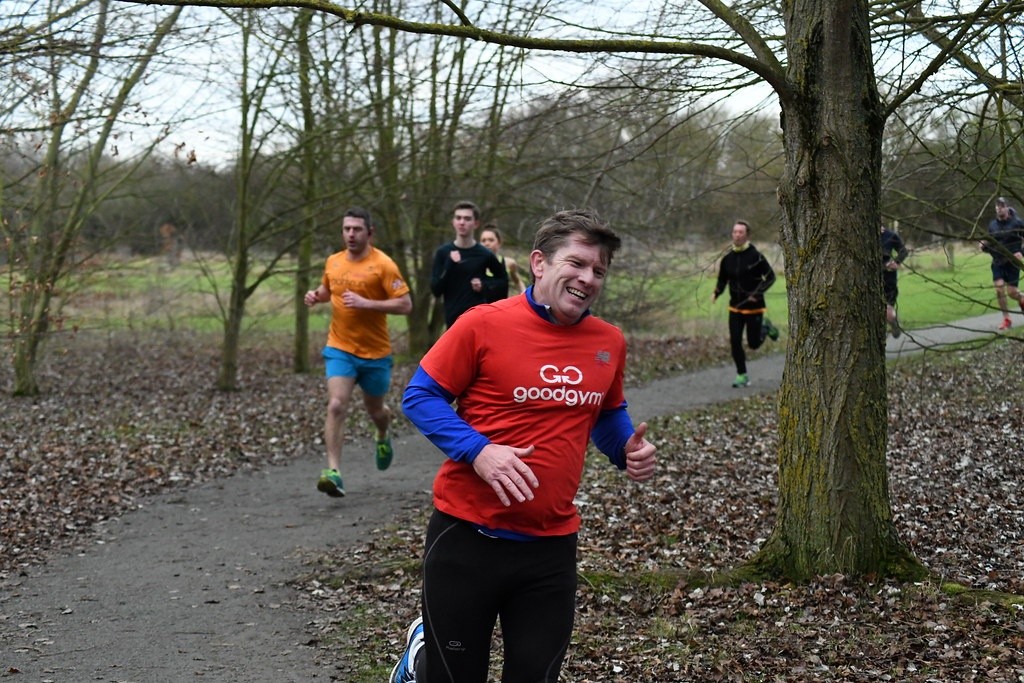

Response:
(375, 428), (394, 470)
(999, 317), (1012, 330)
(762, 314), (779, 341)
(732, 373), (750, 389)
(317, 467), (349, 499)
(386, 614), (431, 683)
(888, 319), (900, 338)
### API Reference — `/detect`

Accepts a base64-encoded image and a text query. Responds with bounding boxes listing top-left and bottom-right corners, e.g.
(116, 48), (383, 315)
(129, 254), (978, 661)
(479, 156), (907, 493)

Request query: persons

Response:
(303, 210), (414, 499)
(979, 198), (1024, 329)
(388, 211), (660, 683)
(478, 222), (526, 301)
(430, 201), (508, 330)
(881, 221), (909, 339)
(711, 220), (780, 389)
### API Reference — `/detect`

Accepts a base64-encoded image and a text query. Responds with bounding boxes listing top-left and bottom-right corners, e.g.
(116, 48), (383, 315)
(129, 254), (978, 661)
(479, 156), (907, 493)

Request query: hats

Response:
(996, 196), (1009, 207)
